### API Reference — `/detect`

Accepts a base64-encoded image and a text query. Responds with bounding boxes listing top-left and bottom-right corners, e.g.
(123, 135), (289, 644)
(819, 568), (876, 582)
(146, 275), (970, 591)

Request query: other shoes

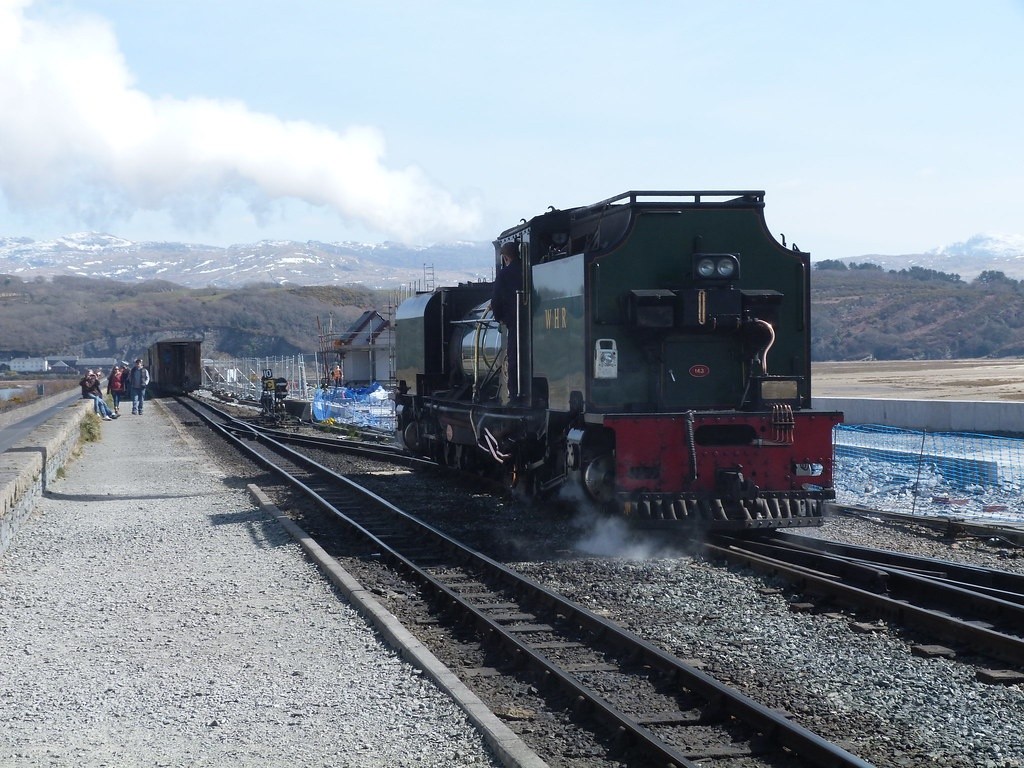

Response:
(115, 410), (121, 417)
(112, 415), (117, 419)
(139, 412), (142, 415)
(102, 415), (111, 421)
(132, 411), (137, 415)
(97, 413), (100, 417)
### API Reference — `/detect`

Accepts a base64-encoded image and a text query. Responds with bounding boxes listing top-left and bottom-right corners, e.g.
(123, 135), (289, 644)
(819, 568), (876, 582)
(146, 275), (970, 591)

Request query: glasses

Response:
(115, 369), (119, 370)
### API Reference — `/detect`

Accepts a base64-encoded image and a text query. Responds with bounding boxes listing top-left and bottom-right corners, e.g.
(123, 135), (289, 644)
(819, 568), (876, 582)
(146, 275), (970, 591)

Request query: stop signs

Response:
(274, 378), (288, 399)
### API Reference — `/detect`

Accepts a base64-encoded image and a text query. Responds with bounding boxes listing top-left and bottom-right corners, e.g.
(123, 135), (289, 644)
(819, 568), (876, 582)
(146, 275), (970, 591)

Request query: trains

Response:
(142, 336), (203, 396)
(390, 189), (846, 531)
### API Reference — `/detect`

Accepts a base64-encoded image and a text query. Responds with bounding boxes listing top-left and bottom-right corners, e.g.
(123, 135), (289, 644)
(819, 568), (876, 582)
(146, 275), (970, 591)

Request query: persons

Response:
(79, 358), (150, 421)
(334, 366), (343, 386)
